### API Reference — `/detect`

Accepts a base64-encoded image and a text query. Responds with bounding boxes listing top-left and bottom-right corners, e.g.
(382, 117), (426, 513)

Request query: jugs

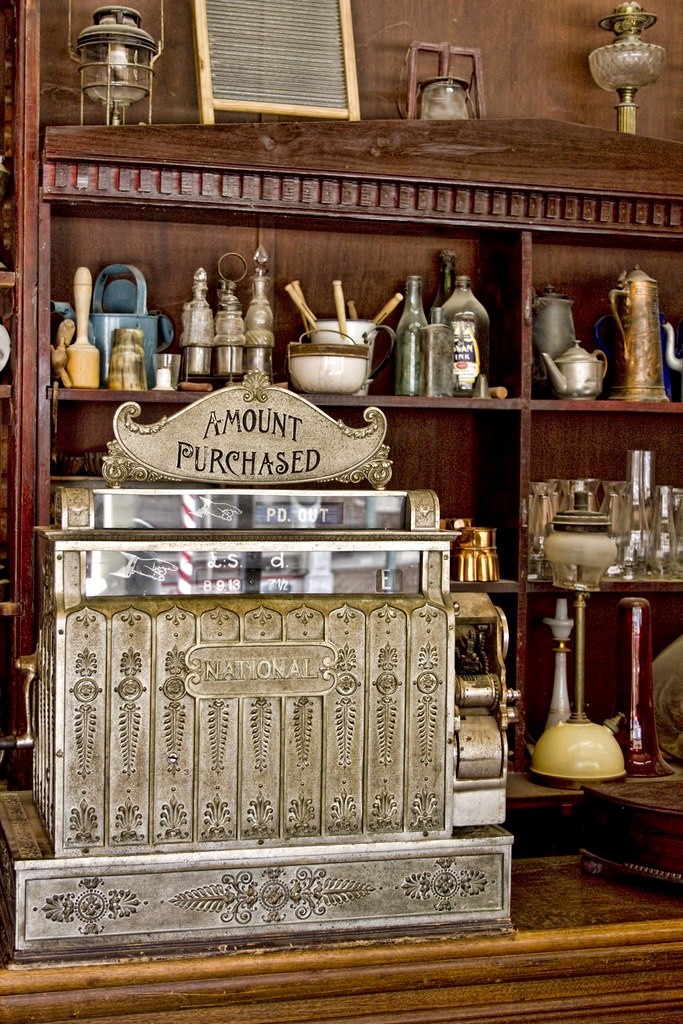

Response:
(50, 264), (174, 387)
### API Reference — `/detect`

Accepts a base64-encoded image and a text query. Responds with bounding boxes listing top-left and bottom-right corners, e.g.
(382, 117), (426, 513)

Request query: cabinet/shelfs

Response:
(0, 0), (683, 858)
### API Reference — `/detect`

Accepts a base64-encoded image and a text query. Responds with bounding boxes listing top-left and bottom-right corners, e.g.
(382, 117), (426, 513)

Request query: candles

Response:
(556, 598), (568, 620)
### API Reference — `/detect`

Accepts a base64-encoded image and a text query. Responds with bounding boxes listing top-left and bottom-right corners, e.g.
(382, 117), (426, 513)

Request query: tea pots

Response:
(542, 340), (608, 400)
(662, 322), (683, 373)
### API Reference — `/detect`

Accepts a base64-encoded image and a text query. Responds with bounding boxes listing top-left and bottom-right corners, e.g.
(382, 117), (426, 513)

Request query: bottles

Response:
(442, 276), (491, 398)
(626, 450), (683, 576)
(420, 307), (455, 398)
(396, 276), (429, 396)
(528, 479), (563, 581)
(560, 478), (601, 513)
(600, 481), (629, 512)
(431, 249), (457, 324)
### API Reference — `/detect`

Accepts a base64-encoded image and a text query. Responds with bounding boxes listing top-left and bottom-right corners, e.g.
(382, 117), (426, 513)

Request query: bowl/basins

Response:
(287, 329), (369, 395)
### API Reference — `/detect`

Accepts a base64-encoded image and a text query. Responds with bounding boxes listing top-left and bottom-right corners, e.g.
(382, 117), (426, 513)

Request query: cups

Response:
(594, 311), (672, 400)
(309, 319), (395, 395)
(152, 353), (181, 390)
(607, 494), (634, 578)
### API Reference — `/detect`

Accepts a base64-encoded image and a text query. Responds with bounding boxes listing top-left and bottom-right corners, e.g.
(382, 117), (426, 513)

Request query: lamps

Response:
(588, 2), (666, 136)
(68, 0), (165, 126)
(529, 491), (627, 790)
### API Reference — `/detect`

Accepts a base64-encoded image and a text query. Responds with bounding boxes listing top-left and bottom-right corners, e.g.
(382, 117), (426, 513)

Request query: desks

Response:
(0, 856), (683, 1024)
